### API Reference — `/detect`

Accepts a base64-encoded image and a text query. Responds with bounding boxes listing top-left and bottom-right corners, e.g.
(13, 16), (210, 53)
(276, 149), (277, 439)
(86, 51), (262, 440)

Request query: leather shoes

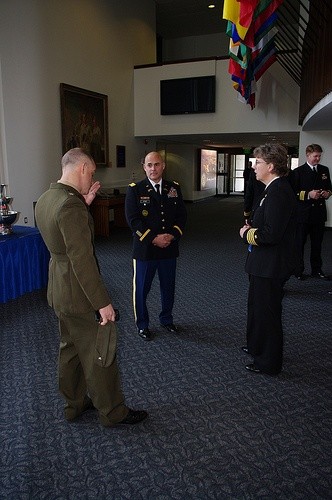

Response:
(159, 322), (176, 332)
(241, 346), (250, 354)
(312, 271), (329, 280)
(246, 363), (263, 372)
(295, 272), (302, 279)
(137, 327), (150, 340)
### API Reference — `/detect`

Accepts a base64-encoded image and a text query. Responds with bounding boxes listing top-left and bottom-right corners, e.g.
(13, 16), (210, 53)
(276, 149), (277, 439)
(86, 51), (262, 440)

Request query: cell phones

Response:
(93, 308), (120, 322)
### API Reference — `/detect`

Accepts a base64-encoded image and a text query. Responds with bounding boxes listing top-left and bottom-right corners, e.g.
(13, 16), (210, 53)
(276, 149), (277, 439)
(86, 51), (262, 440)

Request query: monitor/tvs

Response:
(160, 75), (217, 116)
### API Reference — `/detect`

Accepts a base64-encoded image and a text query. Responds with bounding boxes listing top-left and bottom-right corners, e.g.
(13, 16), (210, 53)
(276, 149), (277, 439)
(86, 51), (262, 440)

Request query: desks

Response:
(0, 226), (51, 303)
(88, 194), (127, 239)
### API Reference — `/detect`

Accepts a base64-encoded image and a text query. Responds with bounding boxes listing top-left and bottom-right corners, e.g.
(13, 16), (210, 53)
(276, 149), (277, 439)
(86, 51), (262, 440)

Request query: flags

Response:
(222, 0), (281, 111)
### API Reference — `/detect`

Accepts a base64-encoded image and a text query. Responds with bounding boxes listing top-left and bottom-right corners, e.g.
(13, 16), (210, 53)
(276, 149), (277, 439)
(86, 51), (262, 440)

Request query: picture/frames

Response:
(59, 82), (109, 167)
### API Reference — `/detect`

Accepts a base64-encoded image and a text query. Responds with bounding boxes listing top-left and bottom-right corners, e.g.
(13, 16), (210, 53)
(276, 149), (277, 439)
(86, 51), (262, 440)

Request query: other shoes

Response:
(76, 398), (98, 420)
(118, 409), (148, 427)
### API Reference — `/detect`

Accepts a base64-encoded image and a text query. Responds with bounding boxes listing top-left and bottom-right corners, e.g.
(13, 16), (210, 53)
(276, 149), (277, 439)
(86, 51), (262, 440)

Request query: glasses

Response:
(255, 160), (269, 166)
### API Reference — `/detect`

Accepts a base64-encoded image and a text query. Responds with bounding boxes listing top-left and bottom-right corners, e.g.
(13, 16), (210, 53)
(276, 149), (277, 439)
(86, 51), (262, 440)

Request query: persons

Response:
(242, 162), (294, 223)
(293, 144), (332, 280)
(240, 142), (305, 375)
(126, 151), (186, 341)
(35, 147), (149, 427)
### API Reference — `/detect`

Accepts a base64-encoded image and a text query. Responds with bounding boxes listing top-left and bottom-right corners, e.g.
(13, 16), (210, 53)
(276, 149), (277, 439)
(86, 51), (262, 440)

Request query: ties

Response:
(313, 167), (316, 173)
(154, 184), (161, 194)
